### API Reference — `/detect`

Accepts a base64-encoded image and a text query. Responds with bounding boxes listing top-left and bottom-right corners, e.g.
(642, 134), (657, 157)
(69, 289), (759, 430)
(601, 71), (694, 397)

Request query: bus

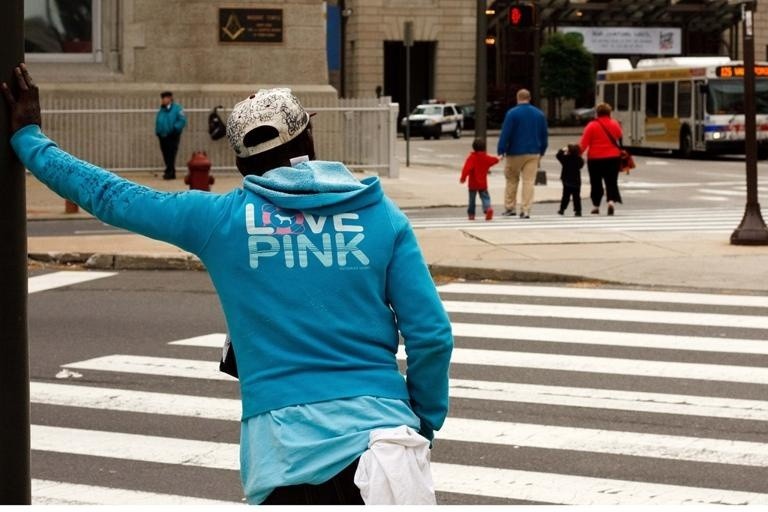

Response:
(592, 56), (766, 159)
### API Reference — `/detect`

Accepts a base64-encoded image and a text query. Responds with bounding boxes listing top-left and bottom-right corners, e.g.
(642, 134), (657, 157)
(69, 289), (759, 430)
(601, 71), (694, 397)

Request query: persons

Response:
(556, 144), (585, 215)
(459, 139), (503, 220)
(3, 63), (453, 505)
(155, 91), (188, 179)
(580, 104), (623, 215)
(498, 88), (548, 219)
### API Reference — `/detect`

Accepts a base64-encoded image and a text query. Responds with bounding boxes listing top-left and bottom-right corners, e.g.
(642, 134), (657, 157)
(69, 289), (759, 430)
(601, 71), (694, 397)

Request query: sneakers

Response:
(468, 214), (475, 220)
(485, 209), (494, 220)
(502, 210), (517, 216)
(590, 204), (614, 215)
(557, 210), (583, 217)
(163, 174), (176, 179)
(520, 212), (530, 219)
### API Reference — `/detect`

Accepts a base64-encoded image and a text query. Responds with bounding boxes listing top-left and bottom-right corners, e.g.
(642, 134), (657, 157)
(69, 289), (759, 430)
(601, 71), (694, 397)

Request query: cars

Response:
(570, 106), (596, 121)
(464, 100), (500, 130)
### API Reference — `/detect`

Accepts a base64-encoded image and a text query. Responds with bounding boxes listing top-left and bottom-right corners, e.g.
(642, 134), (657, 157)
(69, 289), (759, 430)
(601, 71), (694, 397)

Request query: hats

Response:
(161, 92), (172, 98)
(225, 87), (318, 158)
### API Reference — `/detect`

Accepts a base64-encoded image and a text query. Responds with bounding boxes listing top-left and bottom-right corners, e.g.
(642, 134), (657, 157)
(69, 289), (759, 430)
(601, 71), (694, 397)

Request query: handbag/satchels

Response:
(620, 150), (637, 172)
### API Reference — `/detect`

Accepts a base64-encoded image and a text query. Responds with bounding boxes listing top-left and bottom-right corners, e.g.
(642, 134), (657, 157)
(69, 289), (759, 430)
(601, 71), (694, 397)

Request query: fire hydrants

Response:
(185, 152), (214, 189)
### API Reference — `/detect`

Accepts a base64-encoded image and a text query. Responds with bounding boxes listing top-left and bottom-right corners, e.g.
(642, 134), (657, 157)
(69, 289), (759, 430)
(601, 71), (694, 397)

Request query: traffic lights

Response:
(507, 1), (534, 82)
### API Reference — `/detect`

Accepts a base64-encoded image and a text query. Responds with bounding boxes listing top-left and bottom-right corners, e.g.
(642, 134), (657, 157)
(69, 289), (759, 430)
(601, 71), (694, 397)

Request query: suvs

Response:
(401, 98), (464, 141)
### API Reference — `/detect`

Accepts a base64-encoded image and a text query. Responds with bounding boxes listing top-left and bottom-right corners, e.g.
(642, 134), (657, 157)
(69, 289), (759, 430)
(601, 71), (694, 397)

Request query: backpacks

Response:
(208, 105), (226, 140)
